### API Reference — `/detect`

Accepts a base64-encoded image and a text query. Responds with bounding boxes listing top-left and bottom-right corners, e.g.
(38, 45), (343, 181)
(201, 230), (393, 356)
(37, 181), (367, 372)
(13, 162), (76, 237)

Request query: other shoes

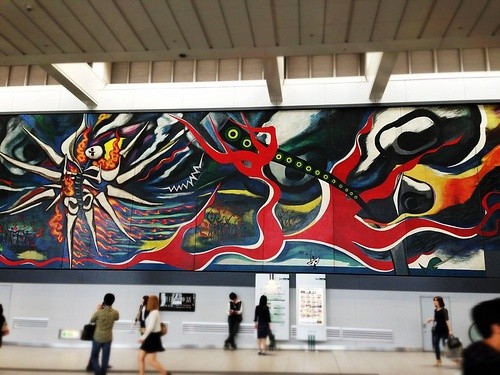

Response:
(434, 360), (441, 366)
(258, 352), (266, 355)
(224, 340), (228, 350)
(231, 345), (237, 350)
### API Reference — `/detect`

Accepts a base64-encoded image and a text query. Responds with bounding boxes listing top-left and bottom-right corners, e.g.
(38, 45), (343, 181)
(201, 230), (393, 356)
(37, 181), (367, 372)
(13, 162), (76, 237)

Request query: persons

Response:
(224, 292), (243, 350)
(461, 297), (500, 375)
(254, 295), (272, 355)
(81, 293), (119, 375)
(426, 296), (453, 366)
(134, 295), (171, 375)
(0, 304), (9, 348)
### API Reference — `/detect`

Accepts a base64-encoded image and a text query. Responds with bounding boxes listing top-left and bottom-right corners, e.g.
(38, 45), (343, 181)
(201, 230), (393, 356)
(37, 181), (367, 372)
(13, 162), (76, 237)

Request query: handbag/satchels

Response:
(81, 319), (96, 341)
(161, 323), (167, 335)
(2, 319), (9, 337)
(448, 335), (461, 349)
